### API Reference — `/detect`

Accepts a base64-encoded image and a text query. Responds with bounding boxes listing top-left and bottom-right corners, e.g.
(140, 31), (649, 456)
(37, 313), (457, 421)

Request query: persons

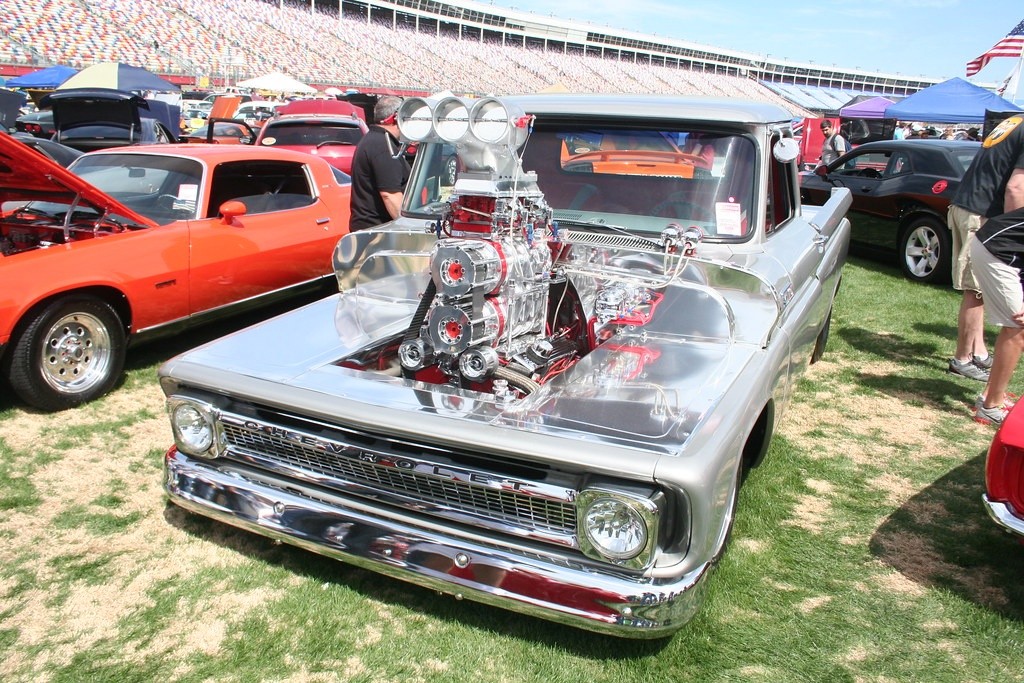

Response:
(949, 113), (1024, 382)
(684, 132), (714, 170)
(970, 206), (1024, 426)
(894, 121), (980, 142)
(820, 120), (846, 170)
(349, 96), (411, 233)
(226, 88), (238, 93)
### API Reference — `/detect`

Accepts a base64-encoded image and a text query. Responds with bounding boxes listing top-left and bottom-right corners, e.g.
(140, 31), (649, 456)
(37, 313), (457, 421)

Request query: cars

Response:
(559, 131), (711, 179)
(797, 138), (983, 285)
(0, 89), (457, 210)
(792, 117), (903, 175)
(0, 130), (353, 412)
(928, 127), (969, 141)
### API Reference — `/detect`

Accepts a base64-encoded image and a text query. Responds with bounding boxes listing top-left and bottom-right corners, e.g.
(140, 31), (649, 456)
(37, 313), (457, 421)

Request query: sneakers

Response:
(975, 403), (1010, 425)
(949, 352), (989, 382)
(972, 393), (1015, 411)
(972, 354), (993, 369)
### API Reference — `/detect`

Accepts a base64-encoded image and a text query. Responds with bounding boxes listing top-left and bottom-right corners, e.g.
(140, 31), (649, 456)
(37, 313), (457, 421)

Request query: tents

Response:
(882, 77), (1024, 140)
(839, 96), (897, 139)
(4, 66), (76, 89)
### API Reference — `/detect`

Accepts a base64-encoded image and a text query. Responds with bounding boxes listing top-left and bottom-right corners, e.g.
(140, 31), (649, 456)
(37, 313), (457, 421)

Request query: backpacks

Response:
(823, 133), (856, 169)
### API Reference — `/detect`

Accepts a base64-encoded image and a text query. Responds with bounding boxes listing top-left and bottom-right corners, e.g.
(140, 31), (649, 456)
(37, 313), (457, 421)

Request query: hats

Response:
(912, 124), (925, 131)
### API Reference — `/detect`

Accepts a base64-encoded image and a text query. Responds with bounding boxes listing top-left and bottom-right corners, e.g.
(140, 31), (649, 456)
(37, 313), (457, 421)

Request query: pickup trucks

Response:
(156, 96), (852, 641)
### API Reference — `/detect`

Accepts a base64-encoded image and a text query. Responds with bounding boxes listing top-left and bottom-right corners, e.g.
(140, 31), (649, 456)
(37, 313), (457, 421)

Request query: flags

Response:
(966, 18), (1024, 77)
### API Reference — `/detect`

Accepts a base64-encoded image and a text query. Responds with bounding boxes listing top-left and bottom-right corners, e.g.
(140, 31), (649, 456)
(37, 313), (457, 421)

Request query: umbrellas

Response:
(56, 62), (183, 92)
(237, 72), (317, 93)
(325, 88), (340, 95)
